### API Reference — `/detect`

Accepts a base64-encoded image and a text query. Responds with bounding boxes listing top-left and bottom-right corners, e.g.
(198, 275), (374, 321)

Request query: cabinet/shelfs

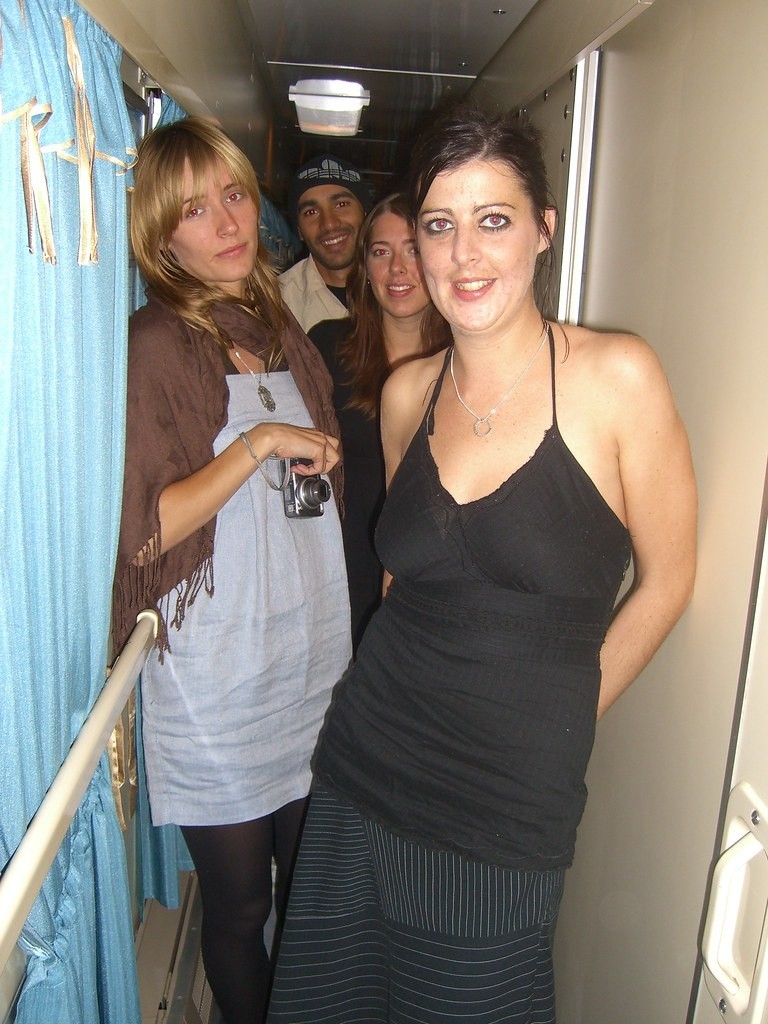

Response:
(299, 133), (405, 175)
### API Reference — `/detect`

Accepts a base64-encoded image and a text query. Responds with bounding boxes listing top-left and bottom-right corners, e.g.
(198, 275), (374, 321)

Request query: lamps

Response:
(289, 77), (371, 137)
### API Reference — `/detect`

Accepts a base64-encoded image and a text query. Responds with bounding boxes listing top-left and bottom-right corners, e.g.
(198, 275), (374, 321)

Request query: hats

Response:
(288, 153), (372, 237)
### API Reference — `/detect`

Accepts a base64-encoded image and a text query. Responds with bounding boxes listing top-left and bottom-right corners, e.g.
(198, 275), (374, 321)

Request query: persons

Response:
(308, 193), (453, 650)
(271, 150), (376, 334)
(111, 116), (358, 1024)
(265, 107), (697, 1023)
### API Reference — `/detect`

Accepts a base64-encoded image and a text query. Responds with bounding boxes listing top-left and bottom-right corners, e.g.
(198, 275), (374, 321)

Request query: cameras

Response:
(280, 457), (332, 519)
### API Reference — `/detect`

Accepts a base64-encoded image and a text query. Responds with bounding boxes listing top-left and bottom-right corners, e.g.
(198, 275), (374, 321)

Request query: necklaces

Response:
(448, 319), (552, 438)
(228, 336), (276, 414)
